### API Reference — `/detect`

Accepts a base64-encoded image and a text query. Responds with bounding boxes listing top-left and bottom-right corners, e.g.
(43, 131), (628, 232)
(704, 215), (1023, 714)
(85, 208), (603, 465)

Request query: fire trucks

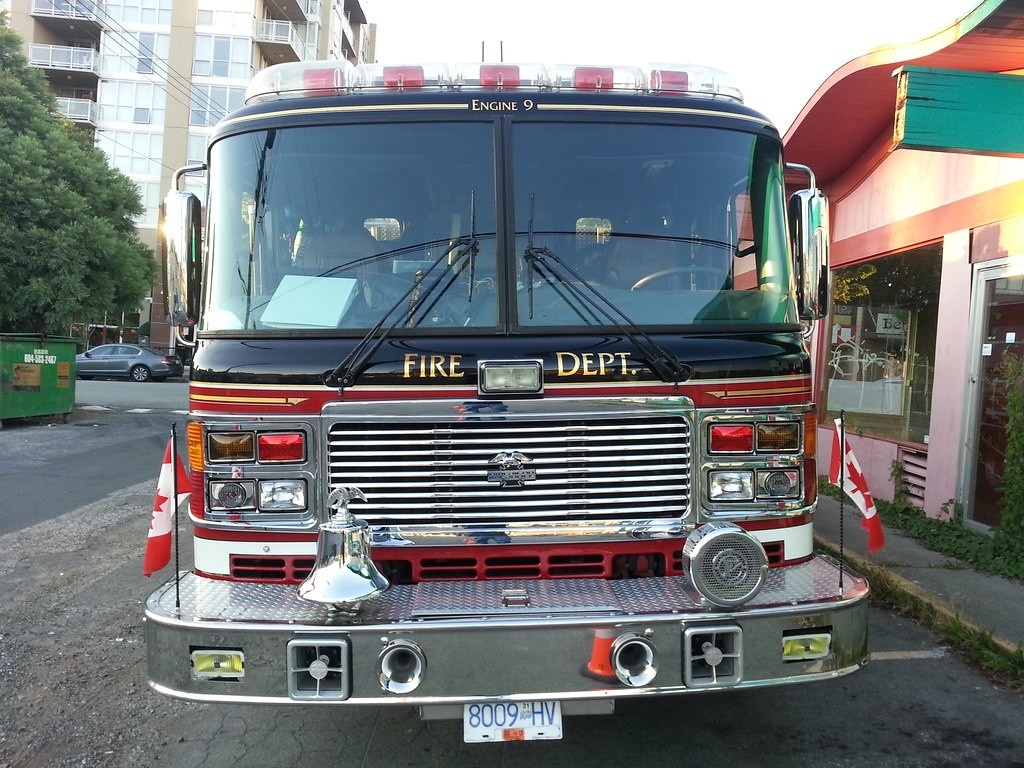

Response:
(145, 40), (885, 745)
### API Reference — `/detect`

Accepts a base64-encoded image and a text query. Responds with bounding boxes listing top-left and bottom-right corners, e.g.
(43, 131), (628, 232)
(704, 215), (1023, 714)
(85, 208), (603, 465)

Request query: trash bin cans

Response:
(0, 326), (83, 428)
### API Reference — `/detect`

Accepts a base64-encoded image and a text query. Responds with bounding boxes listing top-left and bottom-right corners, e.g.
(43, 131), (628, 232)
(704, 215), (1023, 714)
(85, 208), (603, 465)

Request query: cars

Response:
(76, 343), (184, 382)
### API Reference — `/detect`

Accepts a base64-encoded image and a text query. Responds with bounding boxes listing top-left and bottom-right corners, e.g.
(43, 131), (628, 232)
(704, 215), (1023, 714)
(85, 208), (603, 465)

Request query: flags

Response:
(827, 426), (885, 553)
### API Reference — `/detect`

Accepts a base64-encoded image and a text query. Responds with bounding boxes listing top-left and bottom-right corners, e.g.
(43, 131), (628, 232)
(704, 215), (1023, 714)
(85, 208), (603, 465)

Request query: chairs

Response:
(290, 171), (684, 290)
